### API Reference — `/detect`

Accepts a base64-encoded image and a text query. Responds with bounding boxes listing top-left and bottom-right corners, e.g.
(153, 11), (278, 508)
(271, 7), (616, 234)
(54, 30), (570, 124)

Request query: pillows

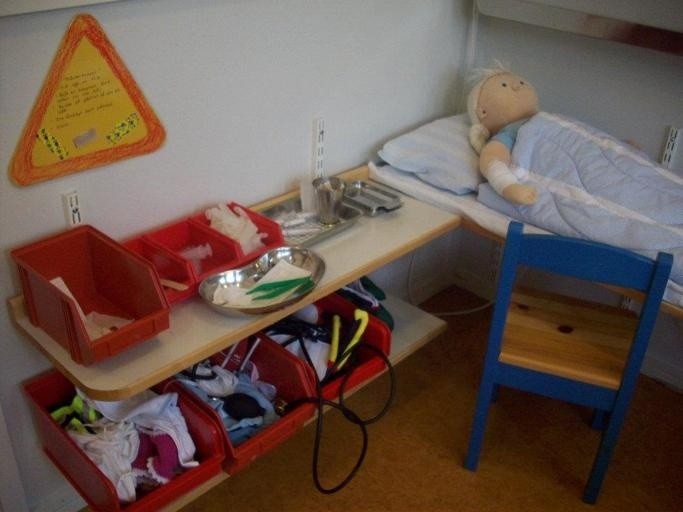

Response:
(375, 108), (485, 198)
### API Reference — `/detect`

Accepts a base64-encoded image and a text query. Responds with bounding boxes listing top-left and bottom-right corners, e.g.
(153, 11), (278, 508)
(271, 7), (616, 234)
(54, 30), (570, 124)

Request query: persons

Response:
(465, 55), (638, 205)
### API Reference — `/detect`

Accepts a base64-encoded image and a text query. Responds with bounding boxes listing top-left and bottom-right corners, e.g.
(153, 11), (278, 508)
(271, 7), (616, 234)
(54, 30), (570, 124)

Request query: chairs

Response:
(464, 217), (674, 504)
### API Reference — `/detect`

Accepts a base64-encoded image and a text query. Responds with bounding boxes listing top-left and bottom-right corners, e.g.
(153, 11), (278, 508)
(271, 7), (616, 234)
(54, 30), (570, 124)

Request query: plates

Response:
(201, 247), (325, 318)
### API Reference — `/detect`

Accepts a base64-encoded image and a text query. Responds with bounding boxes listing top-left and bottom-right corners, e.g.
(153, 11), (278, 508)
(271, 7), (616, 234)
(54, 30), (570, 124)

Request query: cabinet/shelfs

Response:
(5, 163), (463, 512)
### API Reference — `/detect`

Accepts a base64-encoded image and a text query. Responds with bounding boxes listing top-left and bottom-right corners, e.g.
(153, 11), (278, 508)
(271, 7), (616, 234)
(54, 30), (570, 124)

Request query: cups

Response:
(312, 177), (343, 225)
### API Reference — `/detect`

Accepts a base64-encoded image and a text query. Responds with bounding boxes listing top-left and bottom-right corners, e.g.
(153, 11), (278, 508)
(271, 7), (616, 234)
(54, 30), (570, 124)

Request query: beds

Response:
(367, 162), (682, 320)
